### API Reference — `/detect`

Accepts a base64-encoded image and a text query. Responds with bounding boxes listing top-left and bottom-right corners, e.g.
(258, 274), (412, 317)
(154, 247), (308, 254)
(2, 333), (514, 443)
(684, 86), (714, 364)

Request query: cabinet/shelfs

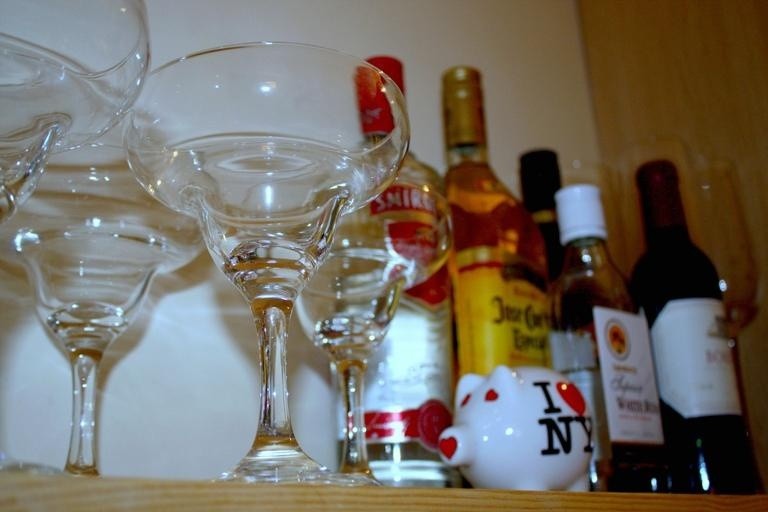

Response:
(0, 1), (768, 512)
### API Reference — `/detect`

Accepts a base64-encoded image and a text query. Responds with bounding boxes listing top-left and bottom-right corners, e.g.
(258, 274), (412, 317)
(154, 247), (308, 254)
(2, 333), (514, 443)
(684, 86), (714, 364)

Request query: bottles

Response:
(441, 66), (553, 489)
(518, 150), (568, 277)
(549, 184), (674, 494)
(332, 55), (452, 483)
(626, 161), (763, 494)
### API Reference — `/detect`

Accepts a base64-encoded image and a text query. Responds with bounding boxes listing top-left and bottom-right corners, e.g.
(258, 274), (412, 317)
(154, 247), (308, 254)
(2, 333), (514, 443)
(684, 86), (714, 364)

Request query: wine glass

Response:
(0, 1), (150, 224)
(296, 178), (454, 486)
(0, 146), (207, 475)
(123, 42), (410, 490)
